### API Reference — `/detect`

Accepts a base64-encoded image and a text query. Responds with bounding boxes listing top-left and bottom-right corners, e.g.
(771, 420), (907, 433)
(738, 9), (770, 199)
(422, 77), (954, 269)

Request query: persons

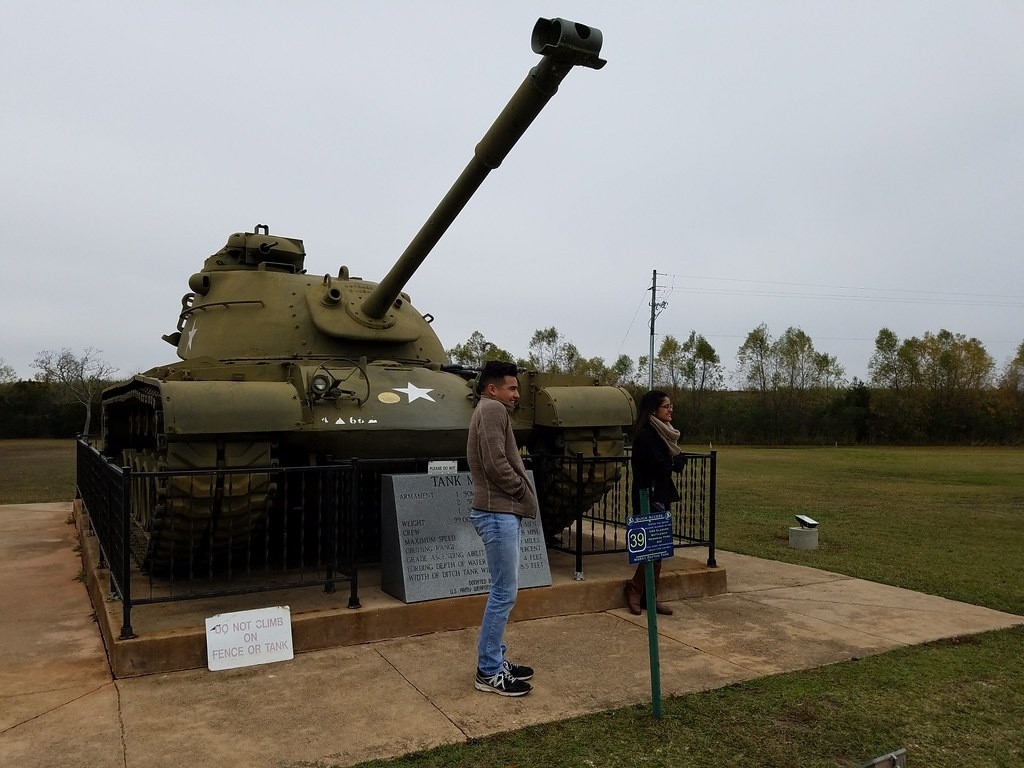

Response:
(466, 362), (538, 697)
(623, 391), (686, 616)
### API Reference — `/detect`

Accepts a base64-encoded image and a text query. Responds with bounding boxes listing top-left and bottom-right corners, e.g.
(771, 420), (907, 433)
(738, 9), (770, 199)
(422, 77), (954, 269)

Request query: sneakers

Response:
(503, 659), (535, 680)
(474, 667), (533, 697)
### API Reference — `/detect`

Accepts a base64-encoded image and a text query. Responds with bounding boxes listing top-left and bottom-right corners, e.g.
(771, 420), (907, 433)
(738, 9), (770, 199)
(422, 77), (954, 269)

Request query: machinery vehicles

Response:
(85, 15), (641, 587)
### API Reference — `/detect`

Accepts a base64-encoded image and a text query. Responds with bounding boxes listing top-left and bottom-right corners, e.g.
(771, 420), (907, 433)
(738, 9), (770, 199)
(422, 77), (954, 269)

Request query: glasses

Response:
(659, 404), (674, 409)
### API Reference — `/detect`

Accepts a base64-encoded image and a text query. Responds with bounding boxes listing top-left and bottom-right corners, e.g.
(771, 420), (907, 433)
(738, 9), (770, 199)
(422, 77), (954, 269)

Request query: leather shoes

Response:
(622, 579), (643, 613)
(641, 597), (673, 615)
